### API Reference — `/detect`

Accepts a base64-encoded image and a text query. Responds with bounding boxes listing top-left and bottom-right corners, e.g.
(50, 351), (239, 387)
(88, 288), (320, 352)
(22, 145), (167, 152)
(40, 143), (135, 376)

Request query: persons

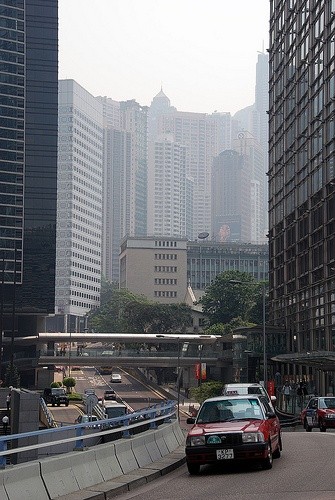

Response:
(118, 347), (122, 357)
(5, 394), (11, 410)
(296, 379), (309, 406)
(282, 380), (292, 405)
(2, 415), (10, 434)
(80, 346), (83, 356)
(55, 347), (66, 356)
(77, 347), (80, 357)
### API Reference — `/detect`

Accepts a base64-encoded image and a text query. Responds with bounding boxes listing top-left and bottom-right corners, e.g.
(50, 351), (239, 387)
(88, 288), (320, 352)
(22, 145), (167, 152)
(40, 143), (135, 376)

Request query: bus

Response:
(102, 351), (115, 358)
(98, 400), (128, 420)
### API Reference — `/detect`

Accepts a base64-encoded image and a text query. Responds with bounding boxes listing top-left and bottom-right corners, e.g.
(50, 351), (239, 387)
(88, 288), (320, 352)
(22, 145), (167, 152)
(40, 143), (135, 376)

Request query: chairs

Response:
(220, 409), (234, 420)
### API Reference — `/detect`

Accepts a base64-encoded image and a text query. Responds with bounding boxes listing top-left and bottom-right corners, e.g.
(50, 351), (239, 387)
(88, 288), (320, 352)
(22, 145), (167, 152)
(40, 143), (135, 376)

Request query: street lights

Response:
(228, 280), (268, 385)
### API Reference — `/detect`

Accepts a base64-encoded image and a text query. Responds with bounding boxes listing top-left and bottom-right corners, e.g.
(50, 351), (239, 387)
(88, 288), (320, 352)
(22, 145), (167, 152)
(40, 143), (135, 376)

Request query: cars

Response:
(299, 397), (335, 433)
(186, 394), (281, 475)
(85, 388), (104, 400)
(104, 390), (116, 401)
(111, 374), (123, 383)
(72, 366), (80, 371)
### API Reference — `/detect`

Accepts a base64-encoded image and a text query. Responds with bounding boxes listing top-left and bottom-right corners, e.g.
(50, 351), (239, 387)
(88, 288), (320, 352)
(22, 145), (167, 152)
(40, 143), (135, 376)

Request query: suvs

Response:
(44, 387), (70, 407)
(96, 366), (113, 374)
(221, 383), (277, 417)
